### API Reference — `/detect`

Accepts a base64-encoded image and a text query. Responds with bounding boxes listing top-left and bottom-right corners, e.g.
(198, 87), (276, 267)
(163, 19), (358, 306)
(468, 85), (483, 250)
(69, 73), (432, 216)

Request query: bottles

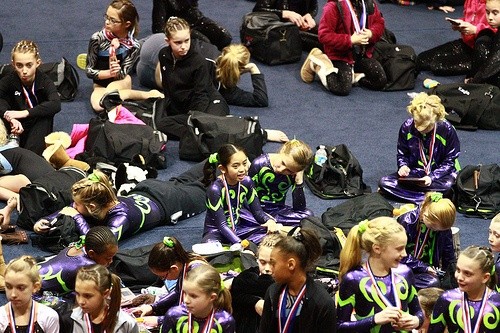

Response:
(314, 145), (327, 167)
(230, 239), (250, 252)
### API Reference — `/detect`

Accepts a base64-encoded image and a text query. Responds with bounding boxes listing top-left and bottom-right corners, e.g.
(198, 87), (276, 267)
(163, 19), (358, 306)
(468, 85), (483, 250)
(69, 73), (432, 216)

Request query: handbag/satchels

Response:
(179, 111), (268, 163)
(83, 118), (167, 169)
(427, 83), (500, 131)
(239, 12), (303, 66)
(38, 57), (79, 103)
(453, 163), (500, 220)
(37, 214), (80, 254)
(370, 28), (419, 92)
(304, 144), (367, 200)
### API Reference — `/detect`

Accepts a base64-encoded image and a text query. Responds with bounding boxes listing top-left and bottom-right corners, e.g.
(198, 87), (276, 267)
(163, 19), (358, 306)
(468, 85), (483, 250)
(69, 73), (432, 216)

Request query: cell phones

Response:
(446, 16), (459, 24)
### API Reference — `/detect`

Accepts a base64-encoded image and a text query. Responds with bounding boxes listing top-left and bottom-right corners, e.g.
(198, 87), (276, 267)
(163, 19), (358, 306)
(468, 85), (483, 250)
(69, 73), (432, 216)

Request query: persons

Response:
(153, 16), (290, 144)
(76, 35), (269, 107)
(300, 0), (386, 96)
(335, 191), (500, 333)
(0, 225), (118, 298)
(418, 0), (498, 75)
(70, 265), (138, 333)
(202, 142), (279, 265)
(464, 0), (500, 86)
(237, 228), (336, 333)
(84, 1), (165, 113)
(152, 0), (232, 50)
(130, 235), (235, 333)
(424, 0), (456, 14)
(0, 255), (60, 333)
(33, 152), (222, 243)
(252, 0), (322, 49)
(377, 90), (461, 209)
(0, 120), (54, 231)
(247, 138), (315, 226)
(0, 41), (62, 157)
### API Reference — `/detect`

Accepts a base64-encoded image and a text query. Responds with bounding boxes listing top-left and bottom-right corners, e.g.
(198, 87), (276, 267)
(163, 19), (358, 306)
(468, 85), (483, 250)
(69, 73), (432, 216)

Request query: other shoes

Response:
(77, 53), (88, 70)
(300, 48), (323, 83)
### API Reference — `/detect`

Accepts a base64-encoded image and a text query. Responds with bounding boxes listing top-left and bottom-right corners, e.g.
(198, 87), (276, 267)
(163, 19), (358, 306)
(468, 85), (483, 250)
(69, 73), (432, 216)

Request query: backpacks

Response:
(322, 192), (395, 237)
(286, 216), (343, 271)
(110, 243), (166, 294)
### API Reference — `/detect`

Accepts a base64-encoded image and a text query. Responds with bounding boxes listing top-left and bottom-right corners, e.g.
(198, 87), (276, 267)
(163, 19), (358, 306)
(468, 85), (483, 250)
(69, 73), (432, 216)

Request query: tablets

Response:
(398, 177), (426, 186)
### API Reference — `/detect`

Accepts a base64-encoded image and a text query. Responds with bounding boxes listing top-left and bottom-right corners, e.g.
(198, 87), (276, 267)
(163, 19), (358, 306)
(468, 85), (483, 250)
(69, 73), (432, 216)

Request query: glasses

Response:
(103, 15), (123, 25)
(159, 272), (168, 281)
